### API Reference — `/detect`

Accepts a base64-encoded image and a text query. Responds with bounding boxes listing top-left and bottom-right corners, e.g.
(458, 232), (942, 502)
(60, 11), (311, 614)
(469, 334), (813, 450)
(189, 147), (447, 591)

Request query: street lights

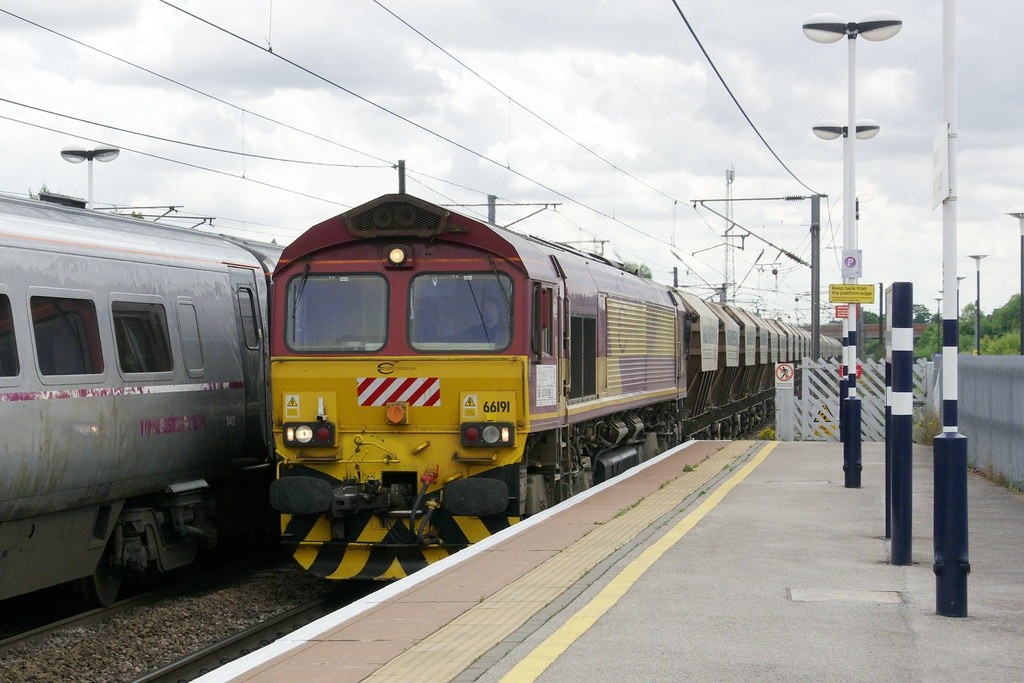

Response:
(934, 297), (942, 354)
(61, 144), (121, 209)
(798, 9), (905, 490)
(808, 117), (883, 443)
(957, 275), (968, 352)
(1004, 212), (1024, 356)
(964, 254), (990, 355)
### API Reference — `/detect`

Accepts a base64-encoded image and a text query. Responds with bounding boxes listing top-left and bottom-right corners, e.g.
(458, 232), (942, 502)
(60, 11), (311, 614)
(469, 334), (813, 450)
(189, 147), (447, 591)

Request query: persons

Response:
(465, 297), (506, 345)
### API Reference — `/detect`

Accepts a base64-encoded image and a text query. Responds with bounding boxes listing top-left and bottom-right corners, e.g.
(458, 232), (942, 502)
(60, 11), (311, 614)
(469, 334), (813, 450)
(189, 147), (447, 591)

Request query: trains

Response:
(260, 188), (840, 583)
(0, 184), (289, 608)
